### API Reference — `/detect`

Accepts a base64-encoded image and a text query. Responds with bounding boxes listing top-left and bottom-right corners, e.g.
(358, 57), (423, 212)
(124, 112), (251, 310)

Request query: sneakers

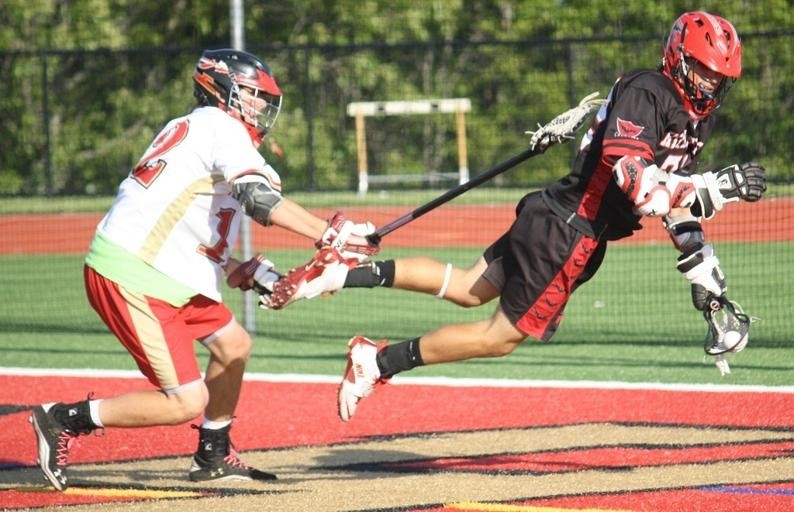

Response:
(269, 247), (350, 312)
(336, 335), (392, 423)
(188, 448), (278, 484)
(28, 400), (80, 492)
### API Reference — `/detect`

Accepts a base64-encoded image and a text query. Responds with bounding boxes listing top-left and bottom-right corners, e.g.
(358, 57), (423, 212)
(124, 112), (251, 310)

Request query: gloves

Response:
(690, 160), (768, 221)
(314, 211), (381, 264)
(676, 241), (728, 312)
(226, 252), (284, 311)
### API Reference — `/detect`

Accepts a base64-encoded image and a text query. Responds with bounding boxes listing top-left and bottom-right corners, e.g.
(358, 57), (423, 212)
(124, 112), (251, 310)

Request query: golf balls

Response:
(724, 331), (741, 348)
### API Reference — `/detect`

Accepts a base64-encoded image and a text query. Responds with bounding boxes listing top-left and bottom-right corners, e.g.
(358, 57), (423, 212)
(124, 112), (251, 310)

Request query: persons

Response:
(20, 46), (379, 494)
(245, 5), (774, 425)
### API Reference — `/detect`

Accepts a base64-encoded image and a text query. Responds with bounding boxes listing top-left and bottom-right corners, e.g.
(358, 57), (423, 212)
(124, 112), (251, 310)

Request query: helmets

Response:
(191, 48), (283, 125)
(661, 10), (740, 86)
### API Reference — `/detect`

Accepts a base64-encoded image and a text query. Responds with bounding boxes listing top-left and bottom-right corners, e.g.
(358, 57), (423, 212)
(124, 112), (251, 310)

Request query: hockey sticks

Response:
(367, 91), (602, 243)
(663, 215), (763, 378)
